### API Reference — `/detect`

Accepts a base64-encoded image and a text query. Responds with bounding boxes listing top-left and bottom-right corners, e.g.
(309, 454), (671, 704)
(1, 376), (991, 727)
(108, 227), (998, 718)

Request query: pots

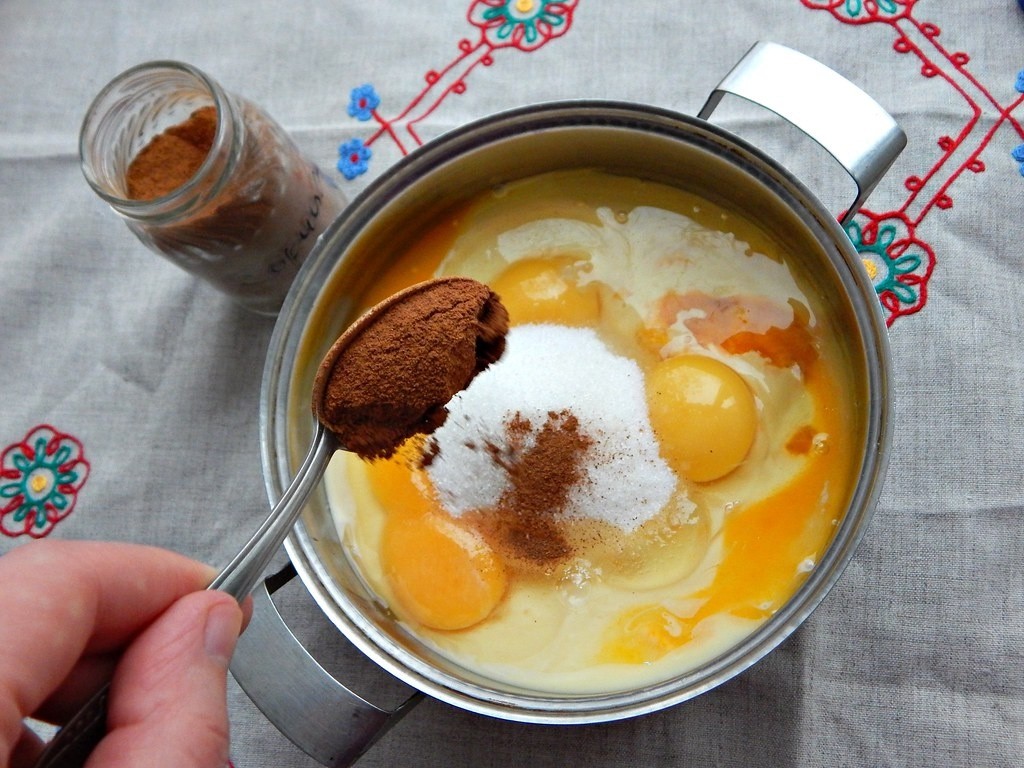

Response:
(228, 40), (906, 768)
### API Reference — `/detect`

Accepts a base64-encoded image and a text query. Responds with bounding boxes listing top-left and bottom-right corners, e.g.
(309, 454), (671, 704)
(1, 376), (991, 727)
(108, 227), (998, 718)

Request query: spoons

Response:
(31, 277), (491, 768)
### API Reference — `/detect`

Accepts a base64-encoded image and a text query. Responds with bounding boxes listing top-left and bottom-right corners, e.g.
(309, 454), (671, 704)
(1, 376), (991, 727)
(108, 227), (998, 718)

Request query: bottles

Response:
(78, 62), (338, 316)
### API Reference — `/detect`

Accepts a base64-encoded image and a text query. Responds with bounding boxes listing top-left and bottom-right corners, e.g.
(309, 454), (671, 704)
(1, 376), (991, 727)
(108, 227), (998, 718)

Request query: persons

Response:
(1, 539), (254, 768)
